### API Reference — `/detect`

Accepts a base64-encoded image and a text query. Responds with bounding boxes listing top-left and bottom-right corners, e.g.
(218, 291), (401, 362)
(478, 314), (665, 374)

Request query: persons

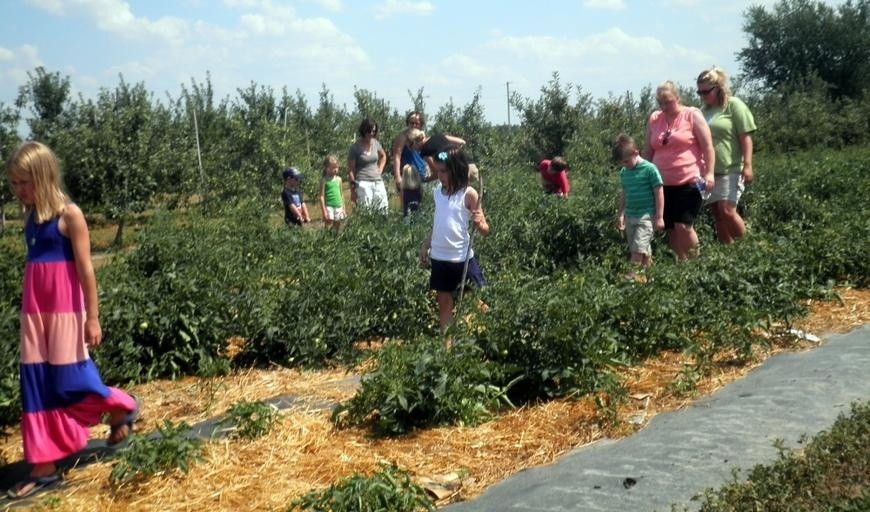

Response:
(347, 118), (388, 220)
(391, 111), (428, 212)
(611, 131), (667, 278)
(695, 66), (756, 249)
(318, 153), (348, 238)
(405, 127), (482, 187)
(396, 162), (424, 219)
(539, 155), (571, 200)
(418, 148), (488, 336)
(281, 166), (311, 228)
(7, 141), (143, 498)
(640, 78), (717, 262)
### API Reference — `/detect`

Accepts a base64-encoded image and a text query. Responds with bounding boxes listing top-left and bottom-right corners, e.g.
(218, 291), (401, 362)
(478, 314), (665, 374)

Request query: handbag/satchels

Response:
(420, 164), (438, 183)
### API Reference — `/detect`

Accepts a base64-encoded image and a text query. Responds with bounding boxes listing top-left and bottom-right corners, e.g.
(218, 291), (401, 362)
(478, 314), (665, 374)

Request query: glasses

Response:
(10, 178), (31, 187)
(662, 127), (671, 146)
(696, 86), (715, 96)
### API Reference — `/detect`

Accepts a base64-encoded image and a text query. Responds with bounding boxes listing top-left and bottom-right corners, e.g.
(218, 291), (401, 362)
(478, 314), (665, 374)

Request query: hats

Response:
(283, 167), (304, 181)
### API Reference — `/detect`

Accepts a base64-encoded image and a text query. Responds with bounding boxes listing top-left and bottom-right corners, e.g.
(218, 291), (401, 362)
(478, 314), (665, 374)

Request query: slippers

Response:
(106, 393), (140, 449)
(7, 473), (61, 499)
